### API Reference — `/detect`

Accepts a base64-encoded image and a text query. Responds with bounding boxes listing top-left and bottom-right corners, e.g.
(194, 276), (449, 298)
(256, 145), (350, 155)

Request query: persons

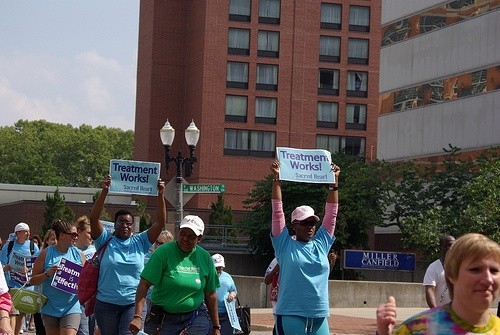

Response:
(211, 253), (239, 335)
(270, 159), (340, 335)
(375, 233), (500, 335)
(263, 235), (337, 331)
(0, 215), (101, 335)
(127, 215), (222, 335)
(140, 230), (174, 330)
(423, 235), (457, 308)
(89, 172), (166, 335)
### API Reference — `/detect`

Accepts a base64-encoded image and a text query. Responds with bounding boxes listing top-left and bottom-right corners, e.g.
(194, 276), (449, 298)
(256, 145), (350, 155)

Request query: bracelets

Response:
(133, 314), (142, 320)
(272, 178), (283, 183)
(328, 185), (339, 191)
(45, 272), (50, 277)
(212, 324), (221, 330)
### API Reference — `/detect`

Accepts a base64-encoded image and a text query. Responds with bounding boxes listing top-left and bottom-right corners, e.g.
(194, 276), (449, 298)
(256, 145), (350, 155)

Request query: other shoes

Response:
(19, 326), (35, 334)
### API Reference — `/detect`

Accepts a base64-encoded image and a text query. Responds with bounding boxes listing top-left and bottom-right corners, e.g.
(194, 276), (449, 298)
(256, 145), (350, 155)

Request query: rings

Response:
(131, 329), (134, 333)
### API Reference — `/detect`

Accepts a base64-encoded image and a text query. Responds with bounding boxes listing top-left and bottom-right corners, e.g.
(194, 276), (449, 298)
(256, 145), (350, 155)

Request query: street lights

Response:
(160, 118), (200, 241)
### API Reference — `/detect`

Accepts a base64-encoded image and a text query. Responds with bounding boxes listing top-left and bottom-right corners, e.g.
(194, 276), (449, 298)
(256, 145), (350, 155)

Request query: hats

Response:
(180, 215), (205, 236)
(15, 222), (29, 232)
(291, 205), (320, 224)
(212, 253), (225, 267)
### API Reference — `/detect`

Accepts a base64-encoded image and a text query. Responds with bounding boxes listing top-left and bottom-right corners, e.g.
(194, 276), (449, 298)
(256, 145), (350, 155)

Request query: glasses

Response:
(115, 221), (133, 226)
(154, 241), (164, 245)
(63, 232), (79, 238)
(294, 220), (315, 226)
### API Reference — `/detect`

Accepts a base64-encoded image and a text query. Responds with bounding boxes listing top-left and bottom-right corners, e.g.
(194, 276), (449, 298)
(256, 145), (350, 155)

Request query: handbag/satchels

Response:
(10, 277), (47, 313)
(62, 232), (112, 317)
(233, 295), (251, 335)
